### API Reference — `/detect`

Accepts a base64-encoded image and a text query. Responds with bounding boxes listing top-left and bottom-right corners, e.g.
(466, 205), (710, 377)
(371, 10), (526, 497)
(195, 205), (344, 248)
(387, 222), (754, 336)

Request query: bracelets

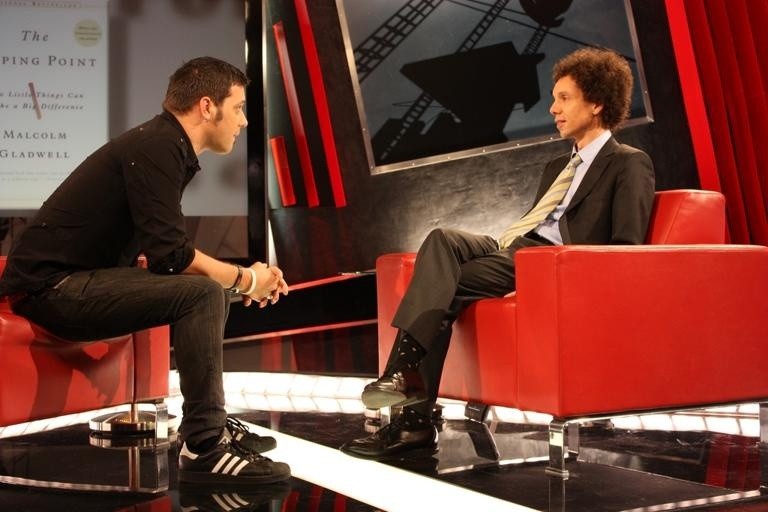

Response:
(239, 268), (257, 296)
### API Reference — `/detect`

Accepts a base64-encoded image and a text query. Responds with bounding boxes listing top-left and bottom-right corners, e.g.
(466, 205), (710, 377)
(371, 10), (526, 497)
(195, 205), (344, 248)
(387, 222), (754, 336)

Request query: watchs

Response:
(224, 264), (243, 294)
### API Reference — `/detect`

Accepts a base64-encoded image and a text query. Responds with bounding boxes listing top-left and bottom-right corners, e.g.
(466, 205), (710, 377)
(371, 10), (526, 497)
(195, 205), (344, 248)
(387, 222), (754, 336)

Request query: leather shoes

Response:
(347, 423), (439, 456)
(378, 457), (439, 479)
(361, 365), (431, 409)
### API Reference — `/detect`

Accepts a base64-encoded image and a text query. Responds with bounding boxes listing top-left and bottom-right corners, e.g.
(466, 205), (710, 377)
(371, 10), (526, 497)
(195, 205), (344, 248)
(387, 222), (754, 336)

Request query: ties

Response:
(498, 152), (583, 249)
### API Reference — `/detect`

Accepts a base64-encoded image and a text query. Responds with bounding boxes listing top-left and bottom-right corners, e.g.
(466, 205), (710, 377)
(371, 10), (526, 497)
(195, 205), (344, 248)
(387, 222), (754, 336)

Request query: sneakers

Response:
(176, 416), (291, 484)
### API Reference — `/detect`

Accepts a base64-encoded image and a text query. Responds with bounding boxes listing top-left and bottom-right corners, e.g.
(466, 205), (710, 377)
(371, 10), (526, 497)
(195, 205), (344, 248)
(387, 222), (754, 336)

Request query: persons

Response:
(340, 45), (656, 458)
(4, 56), (291, 491)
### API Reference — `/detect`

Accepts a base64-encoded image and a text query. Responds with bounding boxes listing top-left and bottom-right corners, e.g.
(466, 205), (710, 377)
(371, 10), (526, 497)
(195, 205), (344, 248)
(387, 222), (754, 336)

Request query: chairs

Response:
(0, 256), (171, 446)
(375, 244), (768, 479)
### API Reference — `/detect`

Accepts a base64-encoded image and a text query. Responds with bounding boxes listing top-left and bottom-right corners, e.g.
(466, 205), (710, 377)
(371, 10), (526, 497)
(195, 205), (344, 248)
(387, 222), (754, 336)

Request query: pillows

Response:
(644, 189), (726, 245)
